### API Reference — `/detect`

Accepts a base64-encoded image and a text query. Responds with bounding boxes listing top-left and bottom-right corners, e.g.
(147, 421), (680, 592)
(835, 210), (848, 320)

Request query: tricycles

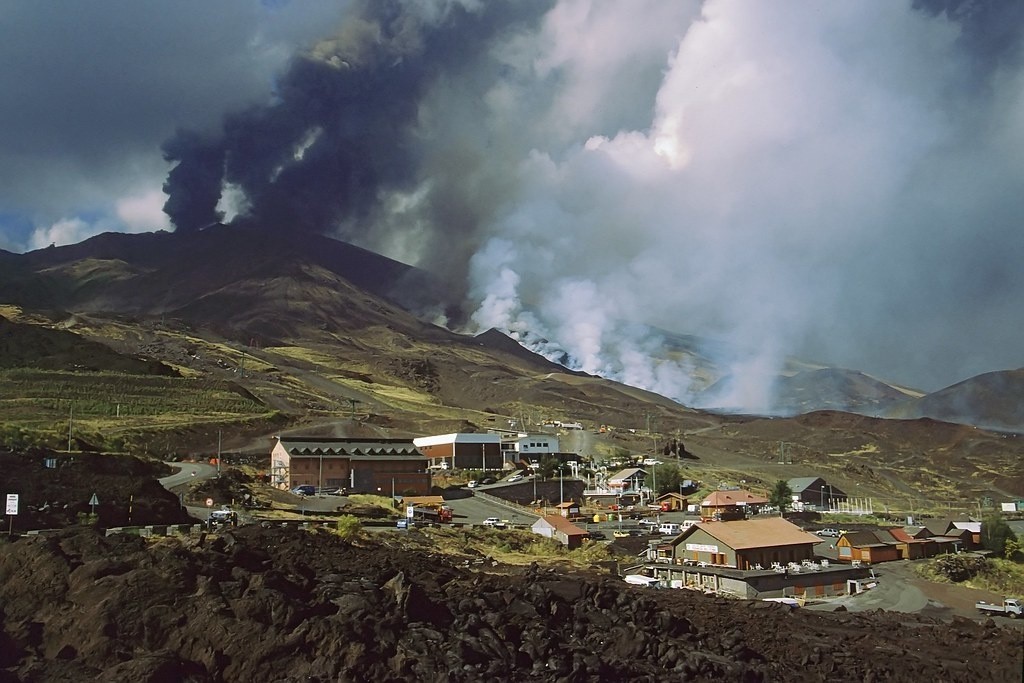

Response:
(439, 505), (453, 523)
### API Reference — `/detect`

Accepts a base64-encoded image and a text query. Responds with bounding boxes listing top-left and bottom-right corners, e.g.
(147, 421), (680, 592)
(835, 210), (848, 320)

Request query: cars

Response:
(292, 484), (314, 494)
(397, 517), (406, 529)
(508, 476), (523, 482)
(594, 426), (612, 434)
(468, 479), (479, 488)
(815, 528), (839, 538)
(482, 478), (496, 483)
(637, 519), (655, 525)
(482, 517), (499, 526)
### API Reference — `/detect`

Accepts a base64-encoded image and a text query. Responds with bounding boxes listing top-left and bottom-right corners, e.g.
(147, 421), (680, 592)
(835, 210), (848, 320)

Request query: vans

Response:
(644, 459), (664, 467)
(658, 523), (680, 536)
(681, 520), (701, 532)
(612, 530), (630, 538)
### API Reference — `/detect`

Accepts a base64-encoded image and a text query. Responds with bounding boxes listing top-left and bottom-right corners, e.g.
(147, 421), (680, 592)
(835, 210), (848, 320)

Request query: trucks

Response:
(431, 462), (450, 469)
(561, 422), (584, 429)
(976, 598), (1024, 619)
(650, 501), (672, 512)
